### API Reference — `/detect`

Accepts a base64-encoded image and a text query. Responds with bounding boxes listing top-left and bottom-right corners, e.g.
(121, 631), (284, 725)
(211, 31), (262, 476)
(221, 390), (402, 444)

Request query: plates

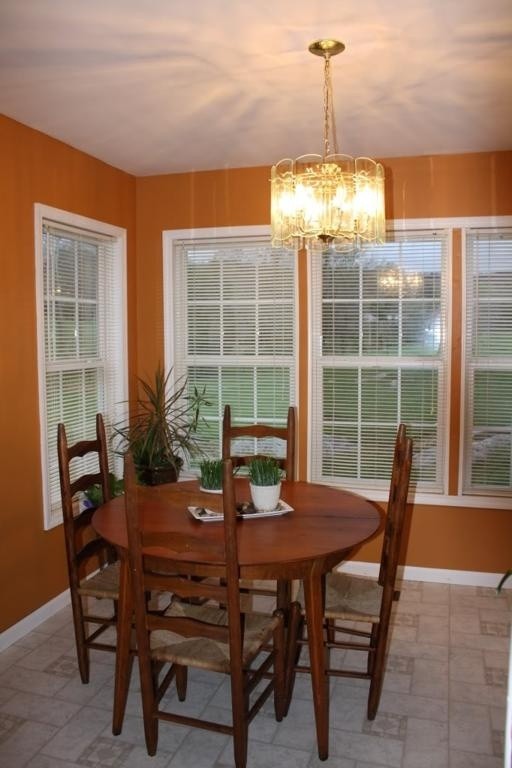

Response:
(187, 499), (295, 522)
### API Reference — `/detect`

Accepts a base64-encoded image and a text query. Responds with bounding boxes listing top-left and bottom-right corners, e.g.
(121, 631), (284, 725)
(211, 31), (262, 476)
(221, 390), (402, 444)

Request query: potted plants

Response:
(199, 459), (223, 494)
(246, 454), (283, 513)
(104, 356), (213, 486)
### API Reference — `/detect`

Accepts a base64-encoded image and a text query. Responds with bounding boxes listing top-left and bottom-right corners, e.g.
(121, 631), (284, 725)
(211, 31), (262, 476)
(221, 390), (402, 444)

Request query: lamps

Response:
(269, 39), (387, 253)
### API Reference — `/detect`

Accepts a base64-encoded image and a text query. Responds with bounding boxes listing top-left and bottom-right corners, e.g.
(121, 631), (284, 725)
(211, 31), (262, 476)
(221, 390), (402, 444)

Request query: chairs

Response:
(124, 452), (285, 768)
(219, 404), (295, 608)
(57, 413), (187, 702)
(286, 424), (413, 721)
